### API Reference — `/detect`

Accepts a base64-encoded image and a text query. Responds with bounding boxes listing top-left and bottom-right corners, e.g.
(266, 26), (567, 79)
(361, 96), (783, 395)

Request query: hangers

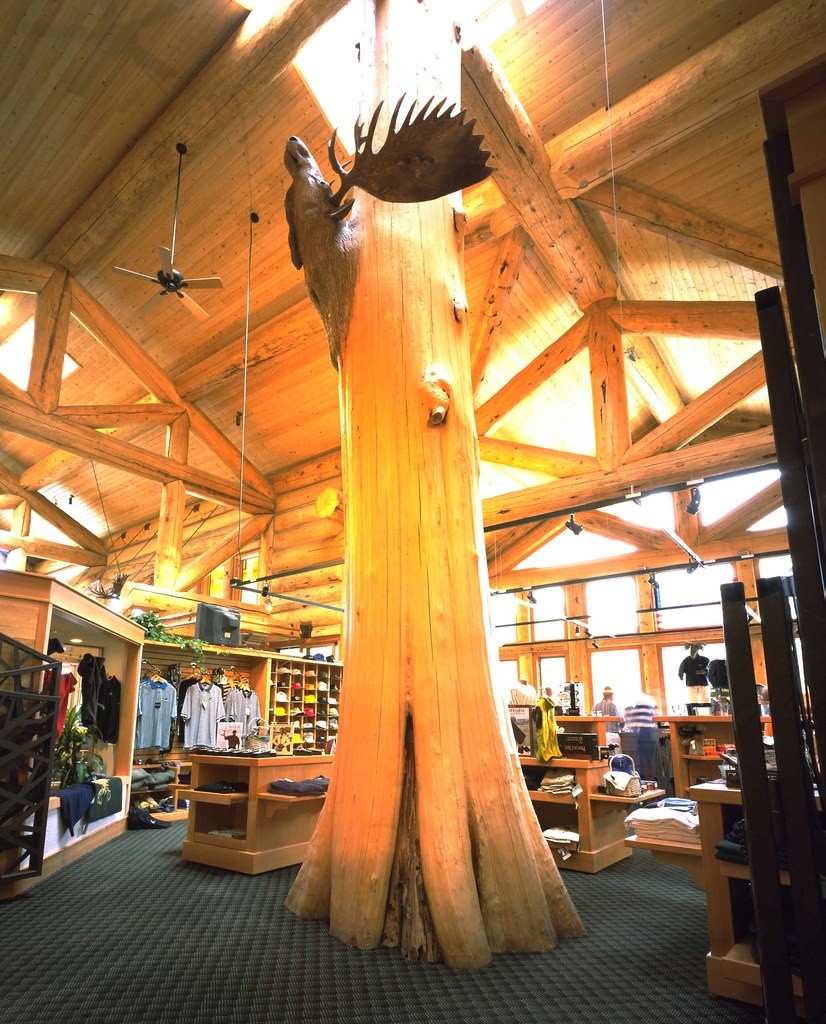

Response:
(179, 670), (222, 693)
(139, 670), (176, 692)
(221, 674), (257, 700)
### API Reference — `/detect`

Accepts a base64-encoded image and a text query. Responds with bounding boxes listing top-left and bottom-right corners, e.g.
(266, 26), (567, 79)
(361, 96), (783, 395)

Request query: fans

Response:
(111, 139), (228, 322)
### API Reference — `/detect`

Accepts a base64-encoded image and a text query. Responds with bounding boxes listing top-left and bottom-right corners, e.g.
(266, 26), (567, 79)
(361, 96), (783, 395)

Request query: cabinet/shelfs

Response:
(133, 640), (270, 823)
(167, 755), (334, 875)
(0, 568), (149, 899)
(651, 715), (777, 801)
(519, 711), (664, 874)
(269, 649), (344, 756)
(623, 778), (825, 1014)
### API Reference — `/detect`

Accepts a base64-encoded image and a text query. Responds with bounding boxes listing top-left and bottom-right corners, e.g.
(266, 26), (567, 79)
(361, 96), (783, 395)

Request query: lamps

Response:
(564, 518), (583, 536)
(685, 485), (703, 516)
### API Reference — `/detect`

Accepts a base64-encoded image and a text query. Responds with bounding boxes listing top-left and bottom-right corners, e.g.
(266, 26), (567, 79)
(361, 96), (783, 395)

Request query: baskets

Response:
(605, 754), (641, 796)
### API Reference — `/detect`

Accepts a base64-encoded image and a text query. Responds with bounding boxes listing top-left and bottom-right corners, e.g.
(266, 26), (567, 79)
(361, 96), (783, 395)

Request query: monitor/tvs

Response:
(196, 602), (240, 647)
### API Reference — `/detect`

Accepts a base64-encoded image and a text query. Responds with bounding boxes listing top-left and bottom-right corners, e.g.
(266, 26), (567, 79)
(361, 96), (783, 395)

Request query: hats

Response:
(601, 687), (614, 694)
(270, 667), (344, 743)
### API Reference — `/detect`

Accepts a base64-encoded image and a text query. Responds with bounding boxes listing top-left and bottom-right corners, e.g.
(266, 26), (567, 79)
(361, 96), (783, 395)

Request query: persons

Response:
(508, 678), (538, 707)
(626, 688), (660, 782)
(677, 641), (710, 715)
(591, 685), (625, 756)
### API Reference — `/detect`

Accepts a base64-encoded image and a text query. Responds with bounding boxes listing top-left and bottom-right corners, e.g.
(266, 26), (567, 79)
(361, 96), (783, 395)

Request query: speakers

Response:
(299, 622), (312, 637)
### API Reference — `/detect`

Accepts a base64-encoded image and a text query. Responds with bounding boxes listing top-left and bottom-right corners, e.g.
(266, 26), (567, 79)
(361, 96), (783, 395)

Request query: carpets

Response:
(2, 810), (761, 1024)
